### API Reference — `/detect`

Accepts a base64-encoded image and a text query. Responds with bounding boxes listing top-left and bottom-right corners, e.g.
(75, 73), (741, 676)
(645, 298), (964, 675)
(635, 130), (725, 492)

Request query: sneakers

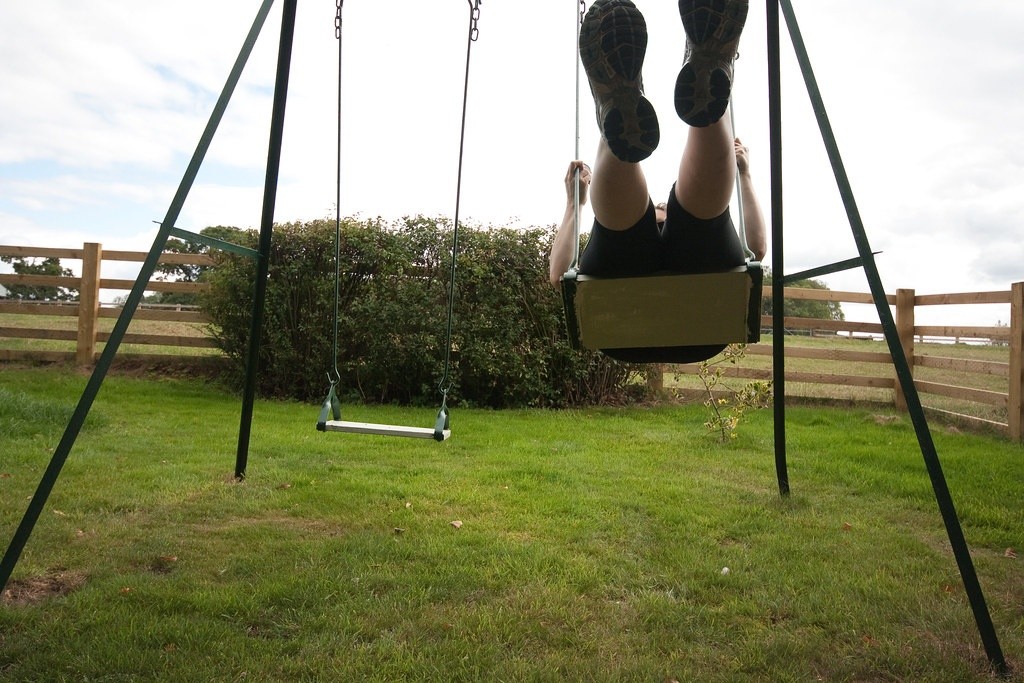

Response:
(674, 0), (749, 128)
(578, 0), (661, 165)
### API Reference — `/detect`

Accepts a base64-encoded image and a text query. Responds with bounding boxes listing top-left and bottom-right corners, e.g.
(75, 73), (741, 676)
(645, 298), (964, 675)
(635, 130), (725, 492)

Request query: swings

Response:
(558, 0), (763, 350)
(312, 1), (480, 440)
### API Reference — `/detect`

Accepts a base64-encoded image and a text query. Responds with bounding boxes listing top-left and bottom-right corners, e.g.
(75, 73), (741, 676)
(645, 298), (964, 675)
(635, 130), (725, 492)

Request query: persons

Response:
(551, 1), (768, 363)
